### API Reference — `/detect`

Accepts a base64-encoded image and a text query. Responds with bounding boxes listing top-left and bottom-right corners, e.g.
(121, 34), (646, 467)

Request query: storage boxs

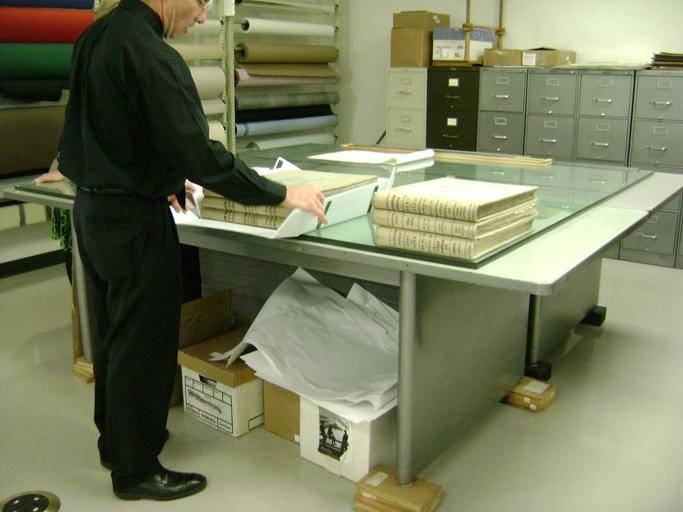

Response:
(477, 111), (525, 156)
(392, 10), (450, 28)
(432, 27), (495, 61)
(299, 395), (397, 483)
(477, 165), (522, 184)
(263, 380), (300, 445)
(425, 161), (476, 180)
(632, 71), (683, 121)
(521, 51), (576, 66)
(527, 69), (579, 115)
(427, 66), (477, 111)
(387, 68), (427, 108)
(573, 190), (608, 211)
(579, 70), (634, 118)
(620, 211), (680, 268)
(393, 168), (424, 187)
(574, 118), (630, 164)
(386, 109), (426, 149)
(628, 120), (683, 165)
(391, 30), (430, 67)
(572, 164), (627, 191)
(426, 109), (478, 152)
(628, 164), (683, 211)
(178, 351), (264, 438)
(523, 185), (572, 210)
(523, 165), (572, 188)
(480, 67), (527, 112)
(524, 113), (576, 162)
(483, 49), (522, 66)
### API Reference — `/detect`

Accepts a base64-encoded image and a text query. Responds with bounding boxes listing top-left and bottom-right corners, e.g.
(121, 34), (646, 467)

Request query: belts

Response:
(77, 185), (138, 197)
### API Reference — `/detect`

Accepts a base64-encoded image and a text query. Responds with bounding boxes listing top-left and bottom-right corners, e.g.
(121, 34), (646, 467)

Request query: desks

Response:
(1, 143), (683, 485)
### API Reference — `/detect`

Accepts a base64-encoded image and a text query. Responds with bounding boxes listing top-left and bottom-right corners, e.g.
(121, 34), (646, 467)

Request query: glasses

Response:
(199, 0), (208, 8)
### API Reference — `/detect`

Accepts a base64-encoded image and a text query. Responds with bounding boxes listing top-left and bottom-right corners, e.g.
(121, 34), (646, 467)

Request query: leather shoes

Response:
(102, 429), (169, 470)
(114, 467), (207, 501)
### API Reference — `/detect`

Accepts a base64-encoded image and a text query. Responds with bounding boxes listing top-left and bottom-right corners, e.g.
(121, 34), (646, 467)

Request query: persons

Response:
(54, 0), (326, 500)
(31, 1), (119, 286)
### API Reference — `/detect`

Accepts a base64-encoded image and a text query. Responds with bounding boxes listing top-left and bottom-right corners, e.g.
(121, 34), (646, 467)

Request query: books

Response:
(201, 163), (377, 231)
(370, 174), (537, 261)
